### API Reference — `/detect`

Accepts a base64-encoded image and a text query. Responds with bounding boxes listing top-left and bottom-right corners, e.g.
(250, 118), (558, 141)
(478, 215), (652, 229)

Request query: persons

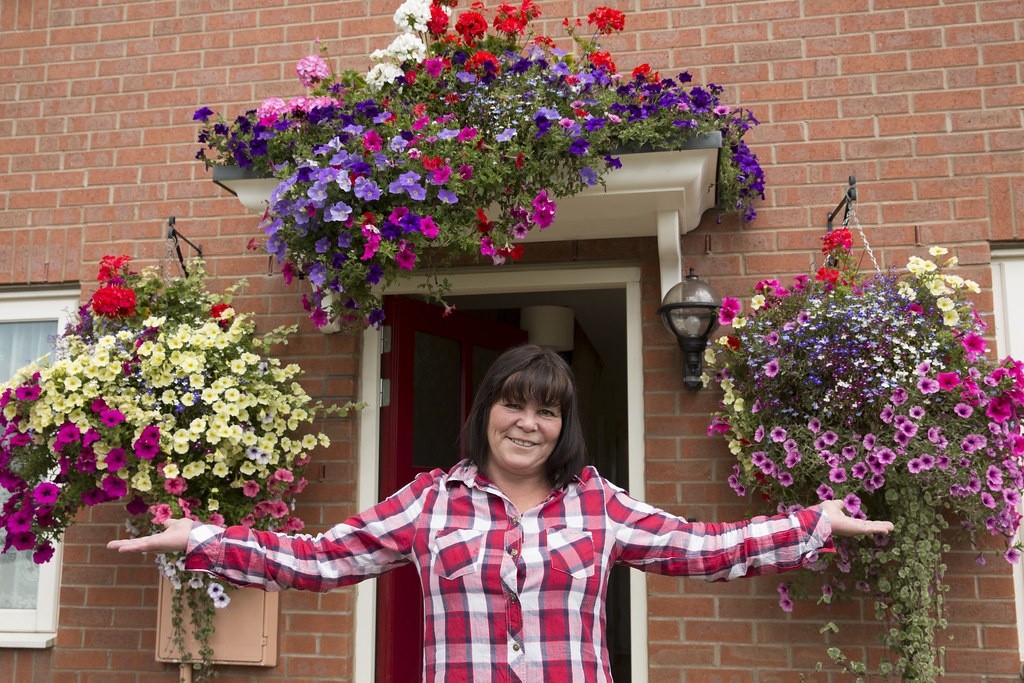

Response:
(103, 343), (893, 683)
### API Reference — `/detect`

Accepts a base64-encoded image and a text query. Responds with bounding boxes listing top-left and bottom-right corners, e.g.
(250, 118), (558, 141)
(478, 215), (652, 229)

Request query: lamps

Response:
(654, 265), (721, 390)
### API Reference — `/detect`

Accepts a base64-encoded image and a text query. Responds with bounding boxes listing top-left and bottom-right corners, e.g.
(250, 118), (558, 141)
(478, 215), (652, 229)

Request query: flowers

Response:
(189, 0), (770, 337)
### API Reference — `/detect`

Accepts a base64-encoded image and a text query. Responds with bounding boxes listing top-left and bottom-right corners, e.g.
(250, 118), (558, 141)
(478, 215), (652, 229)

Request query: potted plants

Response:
(698, 187), (1022, 683)
(1, 225), (368, 683)
